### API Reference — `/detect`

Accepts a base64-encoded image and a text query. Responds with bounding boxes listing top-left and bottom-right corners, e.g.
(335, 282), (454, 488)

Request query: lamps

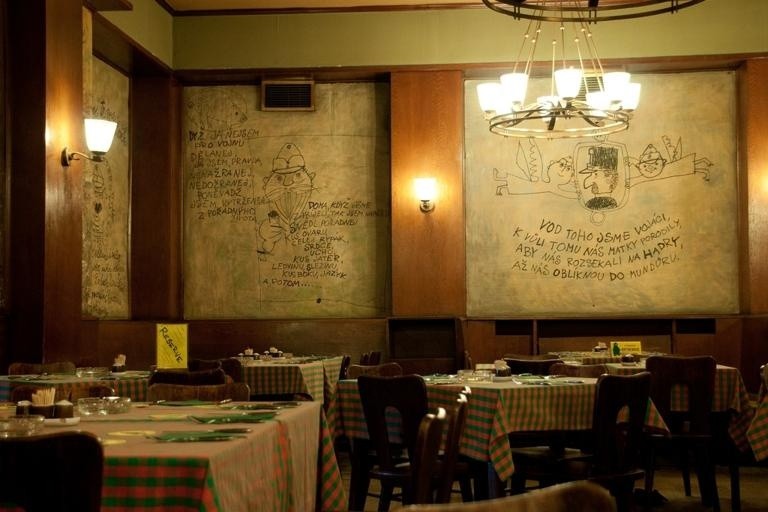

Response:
(60, 114), (118, 166)
(476, 0), (643, 140)
(416, 178), (437, 211)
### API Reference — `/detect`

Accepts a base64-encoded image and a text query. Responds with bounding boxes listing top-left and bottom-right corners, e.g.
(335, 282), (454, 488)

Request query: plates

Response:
(154, 430), (246, 441)
(197, 414), (274, 422)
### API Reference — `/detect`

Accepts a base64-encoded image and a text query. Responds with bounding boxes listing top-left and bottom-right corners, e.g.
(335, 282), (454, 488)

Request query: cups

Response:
(0, 415), (45, 437)
(77, 395), (132, 415)
(457, 368), (493, 384)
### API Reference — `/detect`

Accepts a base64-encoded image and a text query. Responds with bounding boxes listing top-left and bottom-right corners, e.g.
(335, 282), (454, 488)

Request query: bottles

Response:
(612, 343), (619, 355)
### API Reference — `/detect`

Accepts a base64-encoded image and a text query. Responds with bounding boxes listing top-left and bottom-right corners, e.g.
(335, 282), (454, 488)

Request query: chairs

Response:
(0, 430), (103, 512)
(147, 358), (250, 403)
(8, 362), (75, 375)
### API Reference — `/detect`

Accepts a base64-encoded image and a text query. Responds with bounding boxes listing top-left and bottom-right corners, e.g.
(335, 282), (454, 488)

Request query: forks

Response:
(189, 414), (262, 424)
